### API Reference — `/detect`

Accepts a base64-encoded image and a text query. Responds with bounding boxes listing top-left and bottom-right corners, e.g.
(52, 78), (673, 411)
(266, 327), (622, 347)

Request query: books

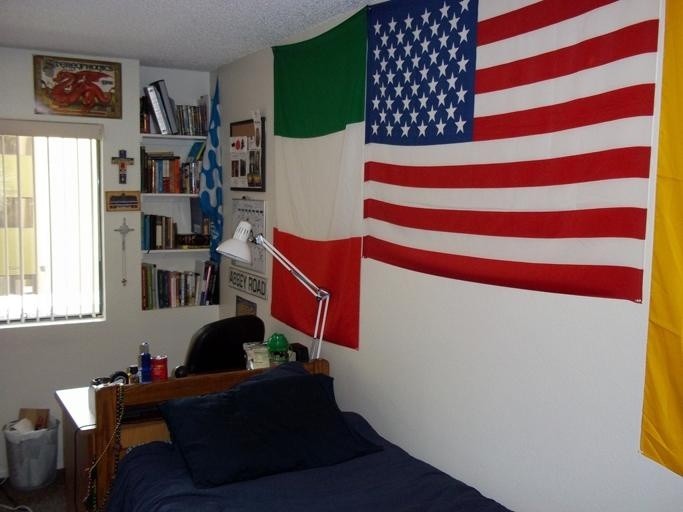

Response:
(138, 79), (220, 311)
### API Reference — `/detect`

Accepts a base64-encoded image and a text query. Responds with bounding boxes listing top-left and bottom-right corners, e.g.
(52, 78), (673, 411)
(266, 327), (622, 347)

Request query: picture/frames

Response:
(228, 115), (266, 193)
(33, 54), (122, 119)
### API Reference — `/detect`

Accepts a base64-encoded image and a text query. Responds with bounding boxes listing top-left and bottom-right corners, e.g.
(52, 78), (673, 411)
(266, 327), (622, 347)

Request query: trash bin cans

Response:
(3, 417), (59, 490)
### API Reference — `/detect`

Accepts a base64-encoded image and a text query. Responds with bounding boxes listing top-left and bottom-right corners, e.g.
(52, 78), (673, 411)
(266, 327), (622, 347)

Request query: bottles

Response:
(268, 332), (291, 370)
(136, 341), (155, 384)
(87, 376), (113, 418)
(151, 355), (168, 384)
(128, 366), (139, 385)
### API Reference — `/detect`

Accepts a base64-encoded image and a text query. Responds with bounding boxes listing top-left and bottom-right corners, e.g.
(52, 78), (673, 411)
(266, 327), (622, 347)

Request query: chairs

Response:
(173, 313), (265, 378)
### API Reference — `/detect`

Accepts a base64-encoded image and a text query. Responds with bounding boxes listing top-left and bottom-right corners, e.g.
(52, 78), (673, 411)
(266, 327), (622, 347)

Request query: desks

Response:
(55, 382), (121, 512)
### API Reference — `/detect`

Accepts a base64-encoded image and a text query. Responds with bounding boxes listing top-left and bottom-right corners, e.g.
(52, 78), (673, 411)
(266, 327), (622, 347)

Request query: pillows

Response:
(153, 372), (384, 490)
(228, 361), (314, 393)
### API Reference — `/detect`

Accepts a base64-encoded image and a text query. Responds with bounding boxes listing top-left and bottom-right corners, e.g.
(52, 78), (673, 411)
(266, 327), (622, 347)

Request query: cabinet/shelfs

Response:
(140, 132), (211, 253)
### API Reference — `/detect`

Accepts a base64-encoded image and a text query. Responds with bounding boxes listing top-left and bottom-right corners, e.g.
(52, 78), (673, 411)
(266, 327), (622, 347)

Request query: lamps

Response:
(213, 219), (330, 361)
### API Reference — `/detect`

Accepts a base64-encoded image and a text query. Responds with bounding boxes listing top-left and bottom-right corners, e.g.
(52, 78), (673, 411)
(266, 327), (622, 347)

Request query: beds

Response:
(86, 356), (514, 512)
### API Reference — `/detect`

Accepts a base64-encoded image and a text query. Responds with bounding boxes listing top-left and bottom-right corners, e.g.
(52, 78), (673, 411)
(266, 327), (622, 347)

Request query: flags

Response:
(635, 1), (683, 478)
(360, 0), (663, 305)
(271, 5), (367, 352)
